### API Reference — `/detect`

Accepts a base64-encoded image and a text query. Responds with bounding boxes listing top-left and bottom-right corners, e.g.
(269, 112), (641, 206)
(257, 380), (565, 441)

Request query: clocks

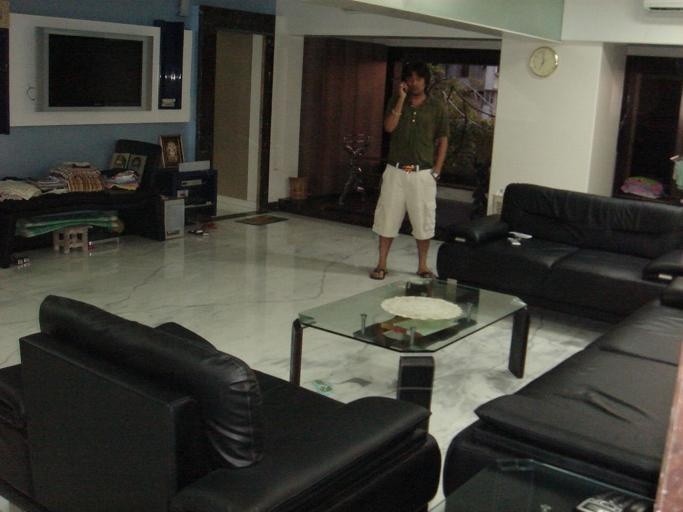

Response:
(526, 46), (559, 79)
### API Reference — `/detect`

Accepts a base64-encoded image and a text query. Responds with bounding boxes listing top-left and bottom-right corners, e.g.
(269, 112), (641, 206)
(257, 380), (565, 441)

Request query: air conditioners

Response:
(642, 0), (682, 16)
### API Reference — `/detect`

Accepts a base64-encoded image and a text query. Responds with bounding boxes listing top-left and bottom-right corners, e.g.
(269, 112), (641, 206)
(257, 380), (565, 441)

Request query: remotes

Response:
(507, 237), (521, 246)
(508, 232), (533, 239)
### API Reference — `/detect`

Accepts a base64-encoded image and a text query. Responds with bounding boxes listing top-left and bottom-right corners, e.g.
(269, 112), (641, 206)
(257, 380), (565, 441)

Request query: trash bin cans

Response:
(288, 176), (310, 200)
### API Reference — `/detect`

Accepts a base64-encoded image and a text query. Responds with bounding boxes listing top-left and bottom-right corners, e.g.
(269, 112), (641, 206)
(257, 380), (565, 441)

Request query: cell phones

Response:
(403, 87), (409, 94)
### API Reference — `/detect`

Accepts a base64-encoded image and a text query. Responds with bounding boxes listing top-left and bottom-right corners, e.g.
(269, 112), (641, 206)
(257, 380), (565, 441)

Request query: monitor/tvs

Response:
(42, 27), (148, 112)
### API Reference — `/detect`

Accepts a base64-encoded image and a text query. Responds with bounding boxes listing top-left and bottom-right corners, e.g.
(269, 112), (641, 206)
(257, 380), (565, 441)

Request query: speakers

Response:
(153, 20), (184, 109)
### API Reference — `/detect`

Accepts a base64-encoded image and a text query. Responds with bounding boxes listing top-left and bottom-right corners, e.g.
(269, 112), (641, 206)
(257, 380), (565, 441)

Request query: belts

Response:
(387, 160), (432, 171)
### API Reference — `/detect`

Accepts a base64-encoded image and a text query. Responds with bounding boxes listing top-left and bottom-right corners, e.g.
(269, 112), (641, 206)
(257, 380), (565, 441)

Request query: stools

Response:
(52, 226), (91, 256)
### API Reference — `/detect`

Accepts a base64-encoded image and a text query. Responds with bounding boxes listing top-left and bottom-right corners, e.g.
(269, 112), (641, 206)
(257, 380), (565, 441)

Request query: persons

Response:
(370, 62), (449, 280)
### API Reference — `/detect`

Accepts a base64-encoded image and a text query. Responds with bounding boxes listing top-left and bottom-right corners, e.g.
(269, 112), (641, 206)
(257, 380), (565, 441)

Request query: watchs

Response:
(431, 170), (441, 182)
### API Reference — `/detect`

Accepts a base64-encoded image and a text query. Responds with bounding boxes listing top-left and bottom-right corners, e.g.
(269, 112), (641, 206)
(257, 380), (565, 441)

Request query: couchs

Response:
(0, 291), (441, 511)
(442, 273), (683, 512)
(435, 182), (682, 325)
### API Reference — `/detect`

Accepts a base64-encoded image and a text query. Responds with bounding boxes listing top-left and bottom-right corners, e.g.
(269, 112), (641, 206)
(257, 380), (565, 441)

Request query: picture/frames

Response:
(158, 133), (184, 170)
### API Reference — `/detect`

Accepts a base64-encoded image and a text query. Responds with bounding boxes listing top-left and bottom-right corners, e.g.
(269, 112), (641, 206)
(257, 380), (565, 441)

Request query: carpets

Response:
(235, 214), (289, 227)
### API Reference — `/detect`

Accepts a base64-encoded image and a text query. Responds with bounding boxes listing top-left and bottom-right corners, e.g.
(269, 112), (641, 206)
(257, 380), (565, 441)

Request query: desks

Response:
(162, 169), (218, 230)
(427, 456), (654, 512)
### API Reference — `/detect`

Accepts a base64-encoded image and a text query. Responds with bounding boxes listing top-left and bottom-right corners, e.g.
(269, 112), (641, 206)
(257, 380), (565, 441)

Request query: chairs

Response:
(0, 137), (162, 268)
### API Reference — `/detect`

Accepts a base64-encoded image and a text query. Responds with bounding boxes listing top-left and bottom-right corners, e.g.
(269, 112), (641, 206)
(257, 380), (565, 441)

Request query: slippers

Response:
(371, 267), (388, 280)
(416, 270), (436, 278)
